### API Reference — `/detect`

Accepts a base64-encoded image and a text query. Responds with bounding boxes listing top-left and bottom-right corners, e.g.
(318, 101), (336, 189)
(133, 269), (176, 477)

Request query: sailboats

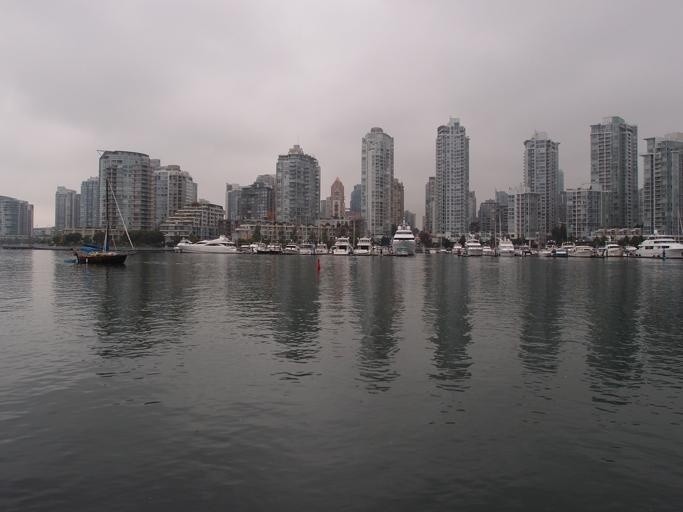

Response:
(73, 178), (128, 265)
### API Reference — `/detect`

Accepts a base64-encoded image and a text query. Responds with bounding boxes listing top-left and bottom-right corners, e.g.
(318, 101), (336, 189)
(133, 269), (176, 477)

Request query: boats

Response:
(173, 235), (237, 254)
(240, 216), (416, 257)
(451, 236), (683, 259)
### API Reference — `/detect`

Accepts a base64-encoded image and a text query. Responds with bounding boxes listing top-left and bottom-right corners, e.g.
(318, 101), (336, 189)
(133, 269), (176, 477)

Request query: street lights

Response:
(487, 216), (496, 246)
